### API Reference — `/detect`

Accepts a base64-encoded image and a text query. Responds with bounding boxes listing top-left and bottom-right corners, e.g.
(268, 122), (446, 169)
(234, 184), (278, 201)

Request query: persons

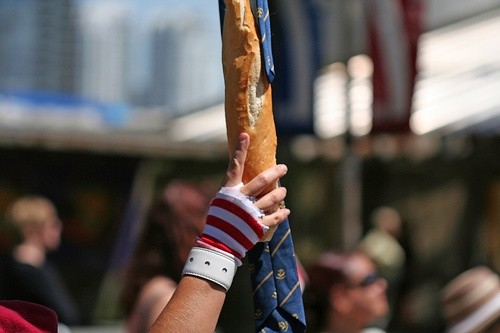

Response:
(0, 158), (500, 332)
(144, 131), (290, 333)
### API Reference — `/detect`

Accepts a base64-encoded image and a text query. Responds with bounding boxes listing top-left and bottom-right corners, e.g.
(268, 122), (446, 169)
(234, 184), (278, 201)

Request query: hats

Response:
(438, 265), (500, 332)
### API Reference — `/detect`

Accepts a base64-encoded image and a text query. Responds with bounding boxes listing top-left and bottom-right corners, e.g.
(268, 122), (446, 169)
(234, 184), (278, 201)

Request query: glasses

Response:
(357, 272), (382, 289)
